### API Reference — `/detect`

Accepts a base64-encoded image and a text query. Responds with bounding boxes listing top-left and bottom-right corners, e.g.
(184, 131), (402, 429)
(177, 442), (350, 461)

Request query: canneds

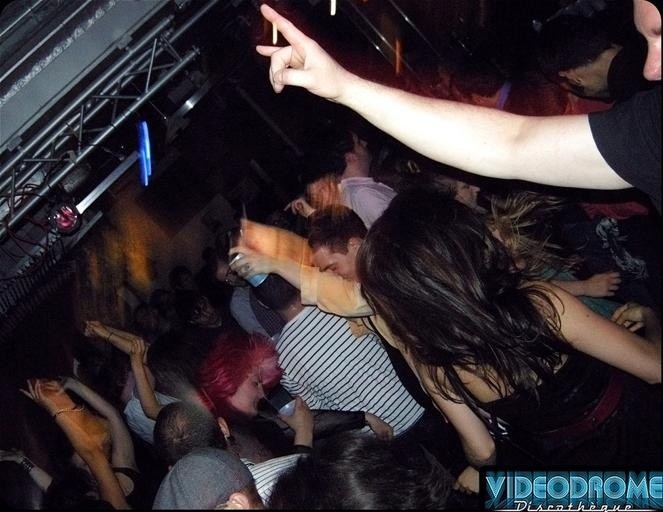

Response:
(279, 399), (296, 416)
(229, 253), (270, 289)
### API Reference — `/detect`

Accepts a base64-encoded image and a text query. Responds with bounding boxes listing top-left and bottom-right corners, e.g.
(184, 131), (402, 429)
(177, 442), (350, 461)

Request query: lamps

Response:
(44, 199), (83, 236)
